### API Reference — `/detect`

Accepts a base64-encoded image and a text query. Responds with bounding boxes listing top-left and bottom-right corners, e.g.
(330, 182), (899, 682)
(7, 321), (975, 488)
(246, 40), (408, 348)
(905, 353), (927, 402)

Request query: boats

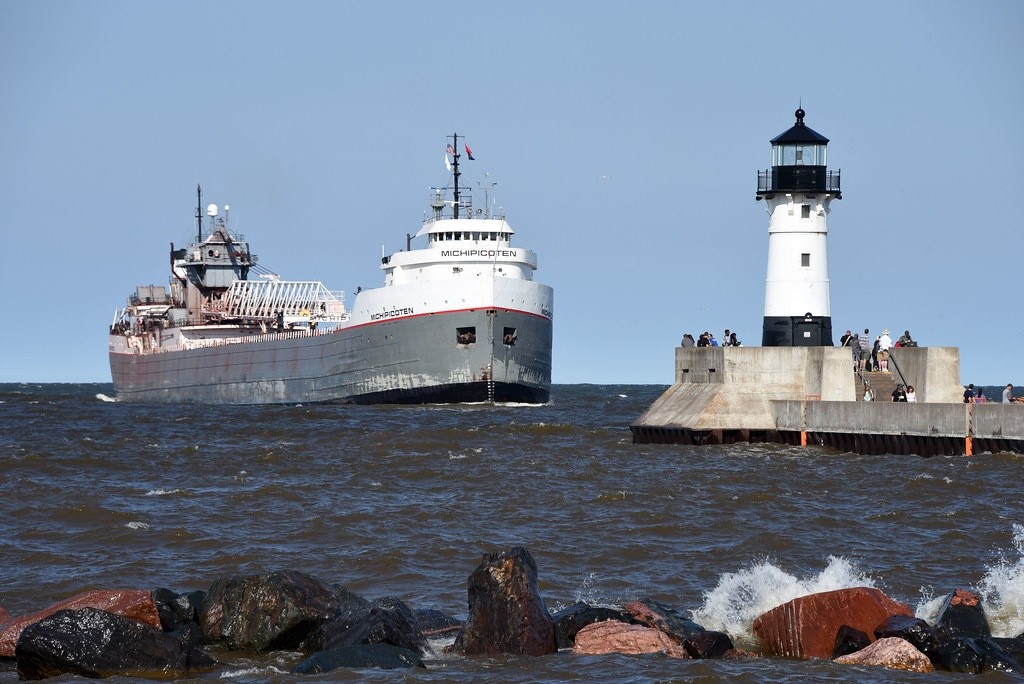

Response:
(108, 132), (553, 404)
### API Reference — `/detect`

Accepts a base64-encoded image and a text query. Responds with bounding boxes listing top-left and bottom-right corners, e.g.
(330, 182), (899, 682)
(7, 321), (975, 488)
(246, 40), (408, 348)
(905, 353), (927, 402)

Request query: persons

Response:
(862, 386), (874, 401)
(1003, 384), (1013, 403)
(973, 389), (986, 404)
(841, 329), (913, 371)
(892, 384), (916, 402)
(697, 332), (718, 347)
(722, 329), (741, 347)
(681, 334), (695, 347)
(963, 384), (974, 403)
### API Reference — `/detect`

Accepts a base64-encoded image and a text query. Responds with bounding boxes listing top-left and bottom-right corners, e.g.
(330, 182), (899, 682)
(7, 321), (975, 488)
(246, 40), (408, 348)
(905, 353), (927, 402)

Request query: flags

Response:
(444, 155), (451, 170)
(464, 144), (474, 160)
(447, 144), (455, 155)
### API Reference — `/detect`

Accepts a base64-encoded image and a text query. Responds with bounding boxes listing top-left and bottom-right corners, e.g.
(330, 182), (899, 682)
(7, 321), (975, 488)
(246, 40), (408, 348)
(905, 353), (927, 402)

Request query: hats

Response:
(897, 384), (904, 388)
(882, 328), (889, 335)
